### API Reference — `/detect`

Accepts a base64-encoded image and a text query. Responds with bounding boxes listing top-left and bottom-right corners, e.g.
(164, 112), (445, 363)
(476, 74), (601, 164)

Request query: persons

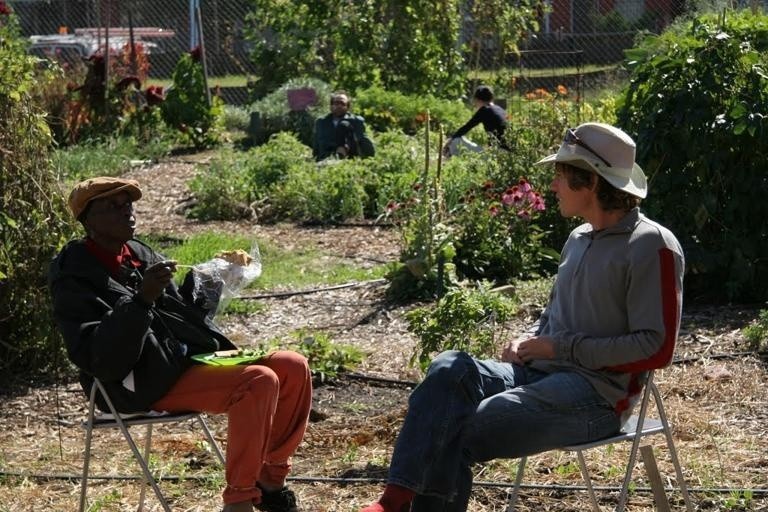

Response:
(353, 122), (686, 512)
(45, 176), (313, 512)
(309, 89), (376, 160)
(443, 85), (513, 159)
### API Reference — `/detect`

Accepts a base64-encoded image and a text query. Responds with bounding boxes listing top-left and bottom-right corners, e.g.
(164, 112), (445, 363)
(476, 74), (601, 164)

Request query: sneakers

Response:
(253, 480), (299, 511)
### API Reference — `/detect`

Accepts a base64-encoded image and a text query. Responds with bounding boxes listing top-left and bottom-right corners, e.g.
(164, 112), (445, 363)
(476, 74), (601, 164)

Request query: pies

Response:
(212, 249), (253, 267)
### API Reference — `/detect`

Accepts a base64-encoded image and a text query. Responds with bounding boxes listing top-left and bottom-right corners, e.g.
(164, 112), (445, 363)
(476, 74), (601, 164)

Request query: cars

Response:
(24, 33), (176, 80)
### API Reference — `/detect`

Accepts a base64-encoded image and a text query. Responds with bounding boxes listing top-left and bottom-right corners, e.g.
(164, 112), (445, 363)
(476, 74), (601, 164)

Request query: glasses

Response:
(564, 129), (610, 170)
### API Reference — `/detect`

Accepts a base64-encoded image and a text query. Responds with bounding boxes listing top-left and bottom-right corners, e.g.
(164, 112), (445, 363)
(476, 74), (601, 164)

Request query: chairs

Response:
(508, 369), (695, 512)
(79, 373), (225, 512)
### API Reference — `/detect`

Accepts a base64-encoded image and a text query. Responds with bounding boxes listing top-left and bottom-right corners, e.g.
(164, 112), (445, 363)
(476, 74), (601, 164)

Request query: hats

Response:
(533, 122), (648, 199)
(68, 176), (143, 220)
